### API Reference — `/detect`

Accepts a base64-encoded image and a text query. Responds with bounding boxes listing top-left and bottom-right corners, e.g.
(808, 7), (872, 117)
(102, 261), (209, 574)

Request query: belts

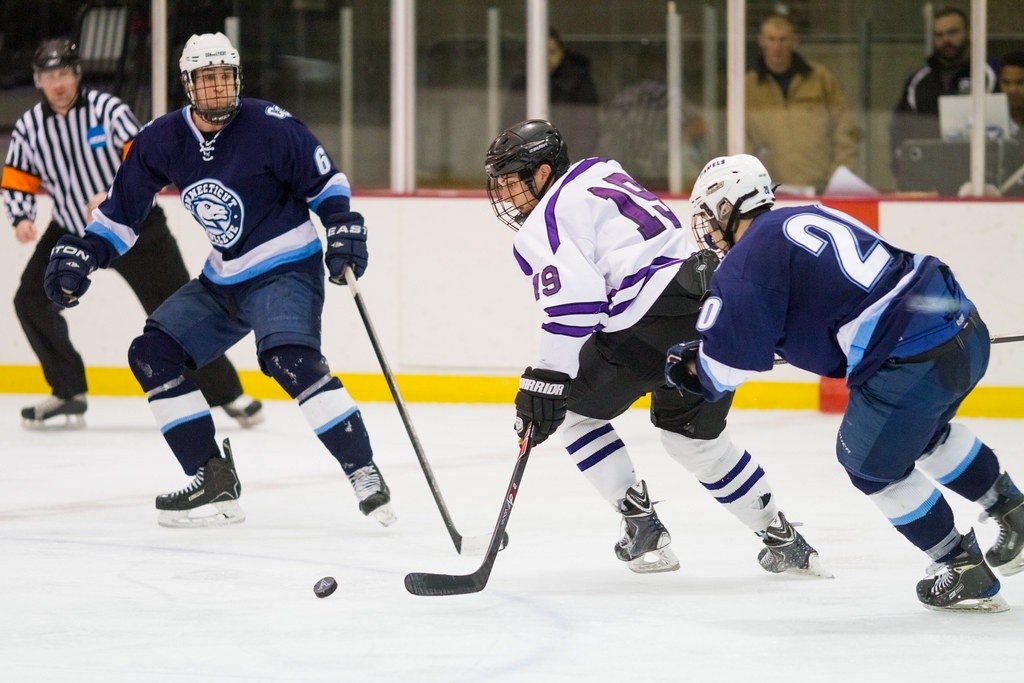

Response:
(889, 306), (978, 364)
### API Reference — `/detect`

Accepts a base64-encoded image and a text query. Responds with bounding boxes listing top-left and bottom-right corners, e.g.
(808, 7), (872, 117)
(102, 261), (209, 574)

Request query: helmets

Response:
(484, 118), (568, 234)
(688, 154), (778, 254)
(32, 36), (78, 70)
(179, 31), (241, 124)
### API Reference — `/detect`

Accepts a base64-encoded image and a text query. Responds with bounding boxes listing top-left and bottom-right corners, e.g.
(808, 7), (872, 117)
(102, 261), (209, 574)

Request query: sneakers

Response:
(19, 395), (87, 429)
(155, 437), (246, 528)
(347, 460), (393, 527)
(757, 510), (835, 579)
(611, 478), (680, 574)
(915, 526), (1009, 614)
(222, 395), (261, 427)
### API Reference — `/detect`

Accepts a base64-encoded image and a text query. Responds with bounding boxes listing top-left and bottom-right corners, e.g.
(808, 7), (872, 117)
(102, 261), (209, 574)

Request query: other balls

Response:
(313, 576), (338, 599)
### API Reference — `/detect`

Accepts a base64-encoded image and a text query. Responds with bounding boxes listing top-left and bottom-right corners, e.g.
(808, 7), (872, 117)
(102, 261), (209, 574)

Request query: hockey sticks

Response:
(344, 263), (511, 557)
(403, 420), (537, 596)
(774, 334), (1024, 367)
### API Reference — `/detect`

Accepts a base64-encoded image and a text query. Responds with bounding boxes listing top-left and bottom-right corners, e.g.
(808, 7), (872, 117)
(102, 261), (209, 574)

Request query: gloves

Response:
(325, 211), (367, 288)
(665, 341), (701, 396)
(43, 234), (96, 312)
(515, 367), (571, 450)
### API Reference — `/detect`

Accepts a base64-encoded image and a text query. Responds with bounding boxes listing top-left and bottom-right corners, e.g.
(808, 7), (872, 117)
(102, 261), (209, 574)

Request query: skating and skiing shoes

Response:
(985, 471), (1024, 575)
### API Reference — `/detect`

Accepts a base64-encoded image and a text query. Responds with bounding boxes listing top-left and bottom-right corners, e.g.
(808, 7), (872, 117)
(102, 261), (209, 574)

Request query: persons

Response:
(1, 39), (263, 422)
(890, 8), (1007, 193)
(745, 12), (861, 196)
(665, 154), (1024, 608)
(44, 32), (391, 517)
(938, 54), (1024, 198)
(502, 26), (599, 164)
(484, 118), (819, 574)
(601, 43), (703, 189)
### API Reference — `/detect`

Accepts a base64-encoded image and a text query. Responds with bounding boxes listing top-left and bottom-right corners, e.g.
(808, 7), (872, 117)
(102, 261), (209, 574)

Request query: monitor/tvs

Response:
(938, 92), (1009, 141)
